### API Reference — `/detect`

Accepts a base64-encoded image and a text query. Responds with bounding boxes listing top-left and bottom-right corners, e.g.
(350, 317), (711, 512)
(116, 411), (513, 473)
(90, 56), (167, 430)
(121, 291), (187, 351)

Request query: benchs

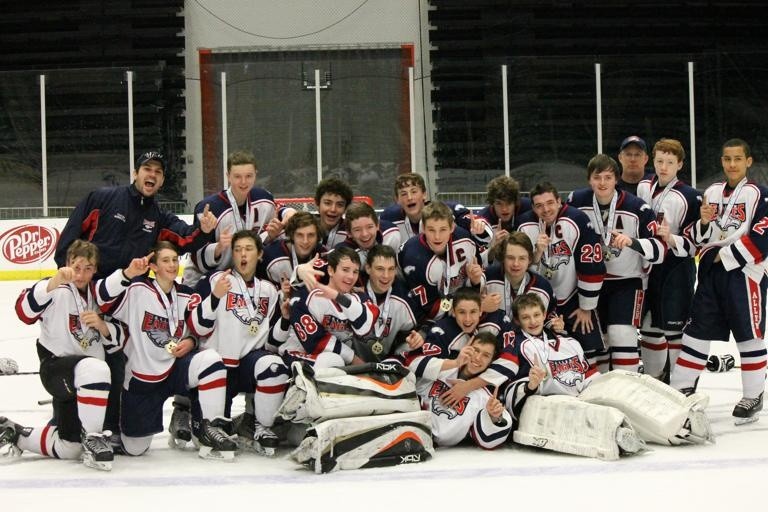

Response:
(0, 0), (184, 178)
(428, 1), (766, 172)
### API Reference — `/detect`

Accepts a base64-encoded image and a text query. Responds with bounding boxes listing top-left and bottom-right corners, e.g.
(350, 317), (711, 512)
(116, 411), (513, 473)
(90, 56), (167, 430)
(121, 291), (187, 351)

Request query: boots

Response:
(732, 395), (767, 419)
(0, 415), (32, 450)
(196, 416), (239, 453)
(238, 415), (280, 448)
(80, 429), (117, 465)
(166, 405), (193, 443)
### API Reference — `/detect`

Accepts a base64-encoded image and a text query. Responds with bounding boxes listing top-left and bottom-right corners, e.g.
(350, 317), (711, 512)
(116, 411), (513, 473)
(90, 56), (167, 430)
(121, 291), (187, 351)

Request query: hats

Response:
(618, 136), (647, 153)
(134, 149), (168, 174)
